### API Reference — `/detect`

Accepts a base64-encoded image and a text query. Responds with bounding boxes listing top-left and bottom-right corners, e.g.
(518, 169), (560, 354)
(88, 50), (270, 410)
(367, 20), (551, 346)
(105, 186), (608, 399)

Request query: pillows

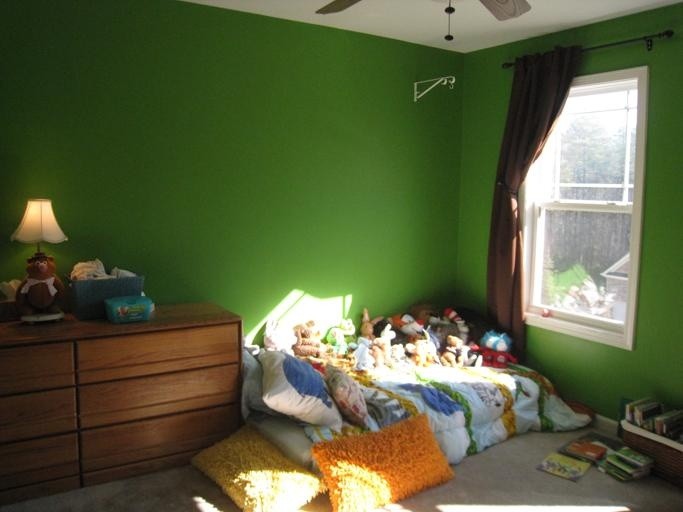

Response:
(311, 414), (454, 512)
(191, 426), (327, 512)
(245, 350), (373, 429)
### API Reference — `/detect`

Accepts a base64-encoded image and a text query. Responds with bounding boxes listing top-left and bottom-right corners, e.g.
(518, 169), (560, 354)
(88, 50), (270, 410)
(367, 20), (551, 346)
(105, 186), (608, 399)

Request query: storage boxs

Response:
(65, 273), (146, 321)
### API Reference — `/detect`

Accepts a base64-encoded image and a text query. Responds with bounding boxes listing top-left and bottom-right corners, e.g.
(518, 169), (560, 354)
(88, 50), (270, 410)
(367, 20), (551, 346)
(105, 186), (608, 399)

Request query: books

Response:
(558, 436), (656, 483)
(621, 396), (682, 443)
(535, 451), (591, 483)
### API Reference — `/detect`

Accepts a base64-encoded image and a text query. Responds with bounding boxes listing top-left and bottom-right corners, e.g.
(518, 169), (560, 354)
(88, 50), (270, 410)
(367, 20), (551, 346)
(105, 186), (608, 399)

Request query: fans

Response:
(315, 0), (532, 21)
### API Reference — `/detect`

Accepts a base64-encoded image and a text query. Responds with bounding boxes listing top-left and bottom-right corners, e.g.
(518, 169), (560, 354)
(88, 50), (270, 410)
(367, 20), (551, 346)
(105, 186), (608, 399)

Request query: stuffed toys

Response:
(292, 302), (518, 378)
(14, 255), (65, 317)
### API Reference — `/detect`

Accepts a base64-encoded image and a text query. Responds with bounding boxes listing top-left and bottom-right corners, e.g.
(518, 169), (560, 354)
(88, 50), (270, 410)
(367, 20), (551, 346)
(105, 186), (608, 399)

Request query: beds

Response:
(248, 360), (598, 474)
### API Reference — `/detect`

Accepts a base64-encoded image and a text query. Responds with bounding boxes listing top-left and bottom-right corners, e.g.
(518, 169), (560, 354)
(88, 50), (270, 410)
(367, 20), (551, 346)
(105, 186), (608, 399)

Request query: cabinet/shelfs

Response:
(1, 301), (244, 508)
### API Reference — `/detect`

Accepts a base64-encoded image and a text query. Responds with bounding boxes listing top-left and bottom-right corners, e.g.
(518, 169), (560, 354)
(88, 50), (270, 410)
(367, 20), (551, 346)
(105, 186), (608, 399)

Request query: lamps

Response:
(9, 197), (69, 325)
